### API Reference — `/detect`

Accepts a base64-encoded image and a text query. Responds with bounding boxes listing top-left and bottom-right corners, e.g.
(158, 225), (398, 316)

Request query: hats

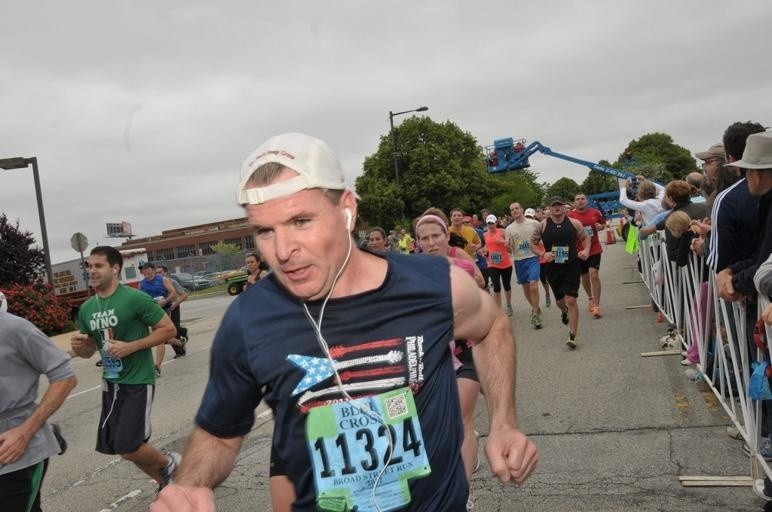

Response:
(722, 128), (772, 170)
(549, 195), (565, 206)
(138, 262), (154, 269)
(695, 143), (726, 160)
(485, 214), (498, 225)
(236, 132), (365, 208)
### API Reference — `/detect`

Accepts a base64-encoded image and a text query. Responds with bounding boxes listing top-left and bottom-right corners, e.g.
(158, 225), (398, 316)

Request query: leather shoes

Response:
(524, 208), (536, 218)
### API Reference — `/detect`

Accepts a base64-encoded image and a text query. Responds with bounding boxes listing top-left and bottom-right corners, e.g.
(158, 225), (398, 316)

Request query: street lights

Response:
(388, 104), (431, 229)
(0, 155), (57, 298)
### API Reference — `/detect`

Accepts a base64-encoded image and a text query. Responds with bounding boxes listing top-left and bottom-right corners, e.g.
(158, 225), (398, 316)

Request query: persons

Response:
(498, 193), (606, 350)
(149, 133), (539, 510)
(2, 292), (77, 511)
(137, 263), (186, 372)
(156, 266), (191, 359)
(605, 120), (771, 511)
(68, 245), (185, 491)
(355, 207), (514, 509)
(242, 251), (275, 291)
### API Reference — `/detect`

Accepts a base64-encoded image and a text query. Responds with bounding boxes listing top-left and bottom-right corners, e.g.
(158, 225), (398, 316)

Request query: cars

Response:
(162, 259), (270, 297)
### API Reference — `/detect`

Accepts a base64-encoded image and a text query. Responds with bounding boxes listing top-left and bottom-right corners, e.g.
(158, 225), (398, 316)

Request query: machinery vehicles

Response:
(482, 136), (667, 219)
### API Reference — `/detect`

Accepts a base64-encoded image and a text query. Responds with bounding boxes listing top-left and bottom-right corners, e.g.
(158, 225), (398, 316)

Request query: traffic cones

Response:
(619, 218), (624, 226)
(604, 219), (616, 245)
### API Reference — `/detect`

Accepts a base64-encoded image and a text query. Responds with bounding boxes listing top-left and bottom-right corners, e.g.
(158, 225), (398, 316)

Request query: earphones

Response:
(345, 209), (355, 231)
(112, 268), (118, 274)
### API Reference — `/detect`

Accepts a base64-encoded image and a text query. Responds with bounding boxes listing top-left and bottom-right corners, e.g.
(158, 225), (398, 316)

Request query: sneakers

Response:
(592, 306), (601, 319)
(545, 293), (551, 308)
(560, 304), (569, 326)
(506, 305), (514, 317)
(727, 426), (772, 503)
(587, 295), (593, 312)
(679, 351), (696, 365)
(157, 450), (182, 495)
(566, 334), (577, 348)
(154, 326), (188, 378)
(530, 308), (542, 327)
